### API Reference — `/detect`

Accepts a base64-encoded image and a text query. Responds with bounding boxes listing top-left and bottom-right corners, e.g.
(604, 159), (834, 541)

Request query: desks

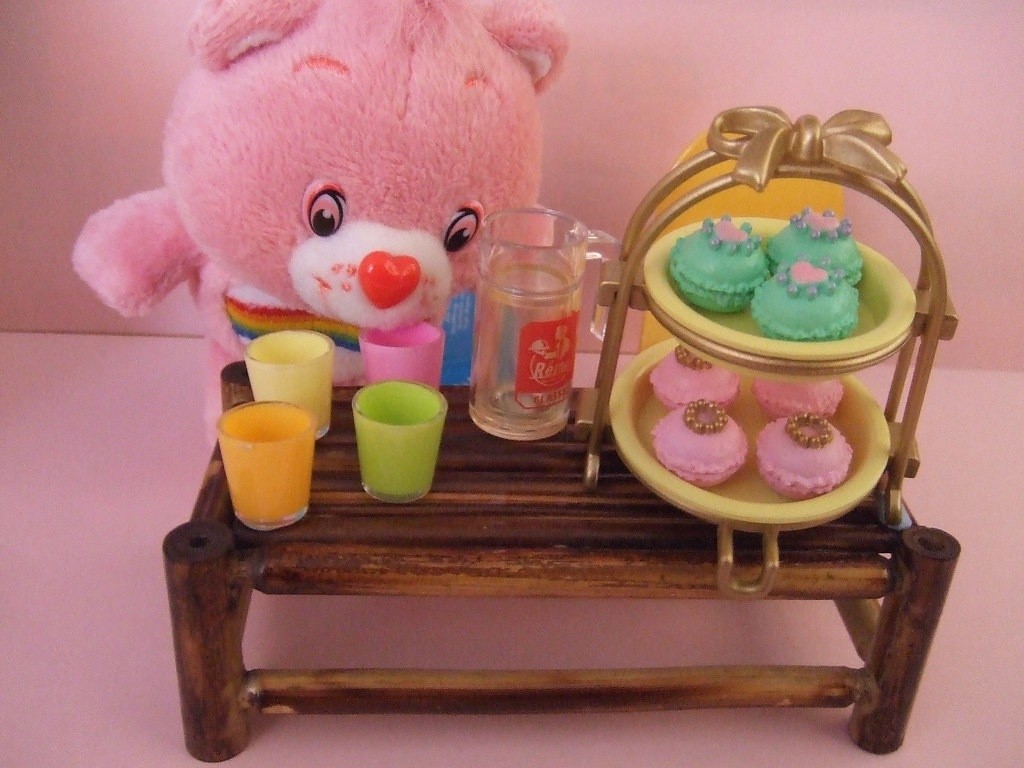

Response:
(161, 362), (961, 763)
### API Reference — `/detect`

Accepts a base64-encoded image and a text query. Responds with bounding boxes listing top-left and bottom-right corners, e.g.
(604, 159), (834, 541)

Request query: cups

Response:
(217, 402), (315, 527)
(244, 330), (334, 438)
(359, 322), (444, 392)
(351, 380), (447, 504)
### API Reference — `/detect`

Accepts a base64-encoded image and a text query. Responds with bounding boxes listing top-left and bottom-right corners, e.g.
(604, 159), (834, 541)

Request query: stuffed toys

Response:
(70, 1), (569, 446)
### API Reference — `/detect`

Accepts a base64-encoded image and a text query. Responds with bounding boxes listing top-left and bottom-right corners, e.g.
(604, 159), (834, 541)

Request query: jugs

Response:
(471, 208), (624, 438)
